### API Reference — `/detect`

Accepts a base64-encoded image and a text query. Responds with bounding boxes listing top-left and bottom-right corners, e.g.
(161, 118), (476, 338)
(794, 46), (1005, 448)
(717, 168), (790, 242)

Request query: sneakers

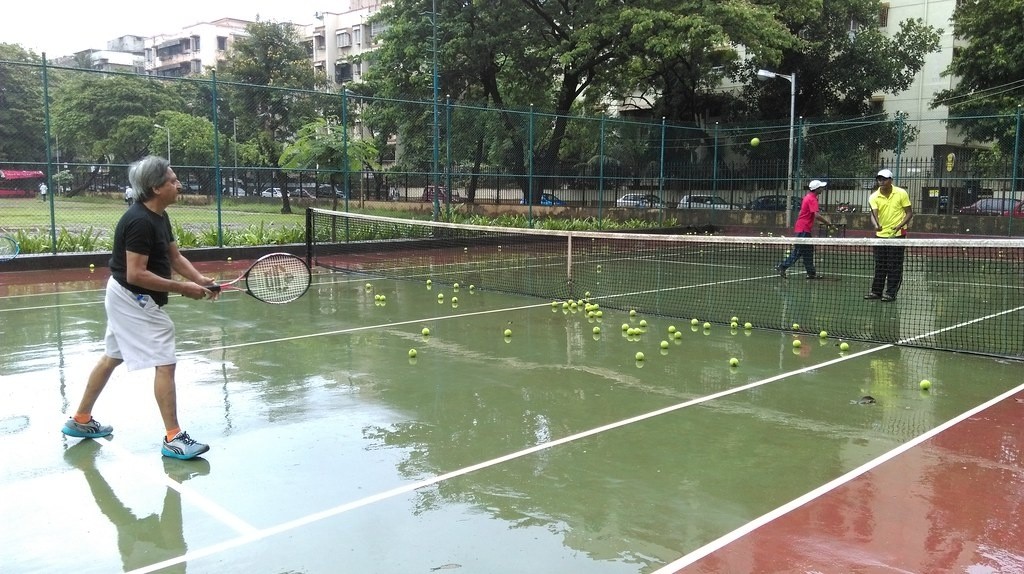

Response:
(62, 416), (113, 437)
(161, 432), (209, 459)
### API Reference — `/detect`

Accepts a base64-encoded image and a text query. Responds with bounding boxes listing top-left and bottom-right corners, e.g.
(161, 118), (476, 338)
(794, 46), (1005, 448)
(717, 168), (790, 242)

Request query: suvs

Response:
(959, 197), (1024, 218)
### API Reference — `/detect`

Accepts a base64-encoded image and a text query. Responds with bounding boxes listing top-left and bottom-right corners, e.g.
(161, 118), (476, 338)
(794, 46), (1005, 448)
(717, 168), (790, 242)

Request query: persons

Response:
(859, 301), (917, 398)
(864, 169), (913, 301)
(772, 280), (821, 357)
(391, 188), (400, 202)
(125, 185), (134, 207)
(59, 156), (223, 462)
(63, 436), (210, 574)
(40, 182), (48, 202)
(777, 180), (836, 279)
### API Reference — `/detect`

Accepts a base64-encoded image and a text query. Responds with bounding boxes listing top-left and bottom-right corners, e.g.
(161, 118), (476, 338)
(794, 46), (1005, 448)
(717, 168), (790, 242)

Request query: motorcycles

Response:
(833, 200), (857, 237)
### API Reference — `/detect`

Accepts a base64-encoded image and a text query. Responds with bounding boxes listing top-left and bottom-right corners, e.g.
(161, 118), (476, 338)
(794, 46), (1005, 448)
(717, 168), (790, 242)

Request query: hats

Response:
(875, 169), (893, 180)
(809, 180), (827, 190)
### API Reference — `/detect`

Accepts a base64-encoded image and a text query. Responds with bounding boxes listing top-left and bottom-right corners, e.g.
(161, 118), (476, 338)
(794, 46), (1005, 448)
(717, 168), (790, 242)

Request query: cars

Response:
(422, 186), (459, 205)
(939, 200), (959, 214)
(614, 193), (668, 209)
(520, 193), (567, 208)
(90, 182), (119, 192)
(744, 195), (804, 211)
(676, 194), (739, 209)
(183, 175), (343, 199)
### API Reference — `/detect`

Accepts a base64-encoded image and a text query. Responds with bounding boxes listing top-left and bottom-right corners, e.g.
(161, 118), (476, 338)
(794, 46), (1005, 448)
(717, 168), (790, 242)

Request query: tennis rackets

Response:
(182, 252), (311, 305)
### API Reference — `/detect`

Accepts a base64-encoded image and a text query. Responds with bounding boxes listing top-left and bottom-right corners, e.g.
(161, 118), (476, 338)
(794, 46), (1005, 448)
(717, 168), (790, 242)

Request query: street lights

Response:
(154, 123), (170, 164)
(44, 130), (61, 196)
(756, 68), (795, 227)
(345, 87), (365, 209)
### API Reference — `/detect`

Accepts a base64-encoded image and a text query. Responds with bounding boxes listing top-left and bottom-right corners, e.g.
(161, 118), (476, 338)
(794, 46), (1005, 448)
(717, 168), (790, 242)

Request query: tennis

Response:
(228, 257), (232, 260)
(829, 229), (835, 234)
(462, 228), (794, 270)
(89, 264), (95, 268)
(90, 268), (95, 271)
(750, 137), (760, 147)
(358, 274), (934, 397)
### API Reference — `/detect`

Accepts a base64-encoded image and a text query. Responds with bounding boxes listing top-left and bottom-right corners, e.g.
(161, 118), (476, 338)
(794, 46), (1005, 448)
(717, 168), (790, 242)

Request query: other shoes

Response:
(806, 274), (823, 280)
(864, 291), (882, 299)
(776, 267), (787, 279)
(881, 292), (896, 301)
